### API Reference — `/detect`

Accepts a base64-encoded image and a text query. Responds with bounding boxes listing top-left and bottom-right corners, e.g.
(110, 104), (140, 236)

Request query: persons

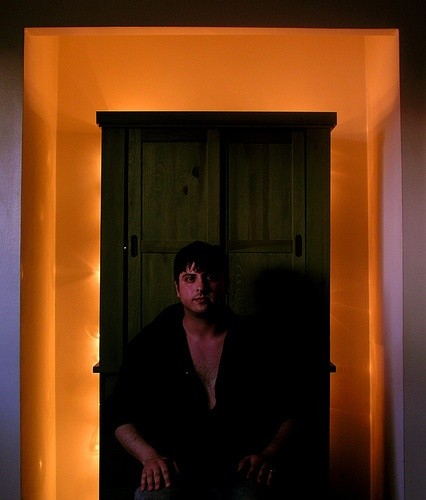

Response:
(108, 241), (295, 500)
